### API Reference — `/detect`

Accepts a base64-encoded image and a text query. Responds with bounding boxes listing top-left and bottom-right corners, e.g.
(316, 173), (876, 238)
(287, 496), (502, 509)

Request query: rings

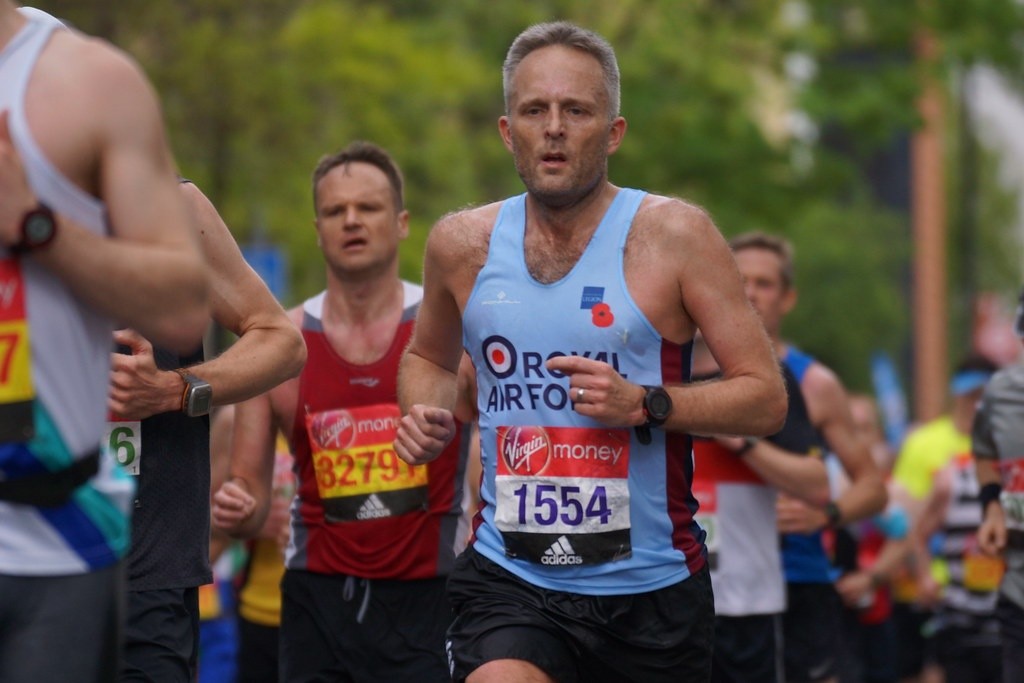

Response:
(577, 388), (585, 402)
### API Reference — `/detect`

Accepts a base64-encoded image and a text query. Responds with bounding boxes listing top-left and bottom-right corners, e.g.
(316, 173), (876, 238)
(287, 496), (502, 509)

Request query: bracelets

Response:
(978, 483), (1002, 508)
(829, 501), (841, 526)
(735, 436), (756, 457)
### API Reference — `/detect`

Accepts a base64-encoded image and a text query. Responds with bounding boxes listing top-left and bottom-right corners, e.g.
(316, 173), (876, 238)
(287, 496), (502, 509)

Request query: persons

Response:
(105, 175), (307, 682)
(689, 326), (831, 682)
(828, 297), (1024, 683)
(392, 23), (788, 683)
(726, 232), (888, 682)
(0, 0), (208, 683)
(196, 144), (481, 683)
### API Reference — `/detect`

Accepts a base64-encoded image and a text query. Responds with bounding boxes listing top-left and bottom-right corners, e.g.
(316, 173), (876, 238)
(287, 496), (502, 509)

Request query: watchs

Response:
(0, 200), (58, 257)
(635, 386), (673, 444)
(175, 367), (213, 417)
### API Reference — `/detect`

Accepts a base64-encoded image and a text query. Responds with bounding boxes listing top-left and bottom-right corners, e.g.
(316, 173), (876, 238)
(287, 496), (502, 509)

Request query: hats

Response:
(949, 370), (994, 394)
